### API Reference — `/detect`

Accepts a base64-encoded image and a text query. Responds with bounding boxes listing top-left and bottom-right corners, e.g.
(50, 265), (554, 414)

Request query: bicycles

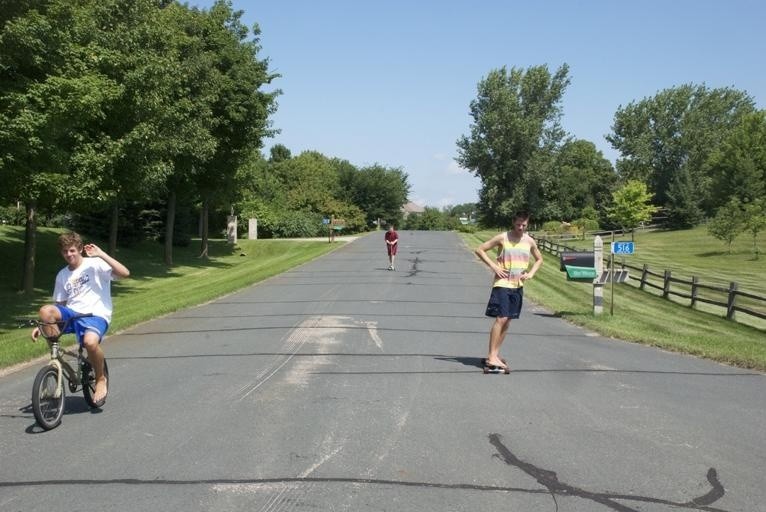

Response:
(14, 312), (110, 431)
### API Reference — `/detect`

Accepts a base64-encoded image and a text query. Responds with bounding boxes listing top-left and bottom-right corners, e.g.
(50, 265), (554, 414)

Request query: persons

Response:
(32, 232), (130, 403)
(475, 211), (543, 369)
(385, 226), (399, 270)
